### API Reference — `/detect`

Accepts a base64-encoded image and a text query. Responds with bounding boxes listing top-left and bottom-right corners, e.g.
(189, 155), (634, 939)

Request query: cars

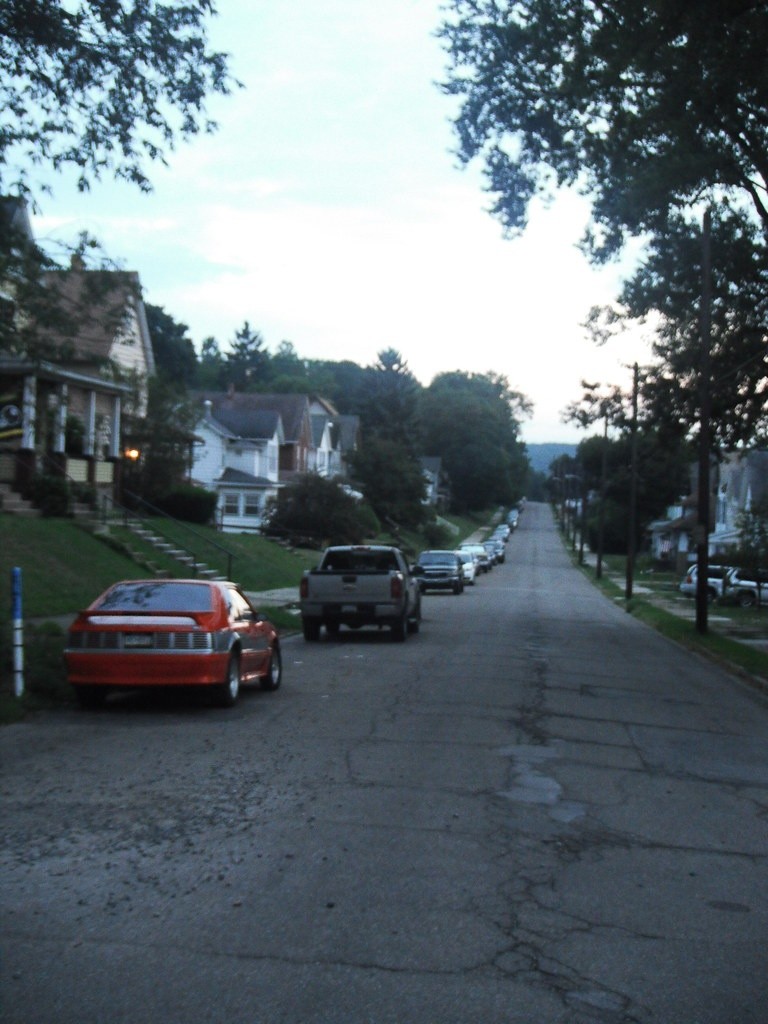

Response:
(65, 578), (287, 703)
(453, 551), (478, 584)
(461, 495), (527, 574)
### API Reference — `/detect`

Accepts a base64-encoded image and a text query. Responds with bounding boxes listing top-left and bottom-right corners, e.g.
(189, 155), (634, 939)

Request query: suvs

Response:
(414, 551), (464, 593)
(722, 567), (768, 607)
(679, 564), (728, 604)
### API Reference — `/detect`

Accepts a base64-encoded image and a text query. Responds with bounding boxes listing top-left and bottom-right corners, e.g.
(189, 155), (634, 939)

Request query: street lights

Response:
(566, 474), (585, 565)
(554, 477), (566, 533)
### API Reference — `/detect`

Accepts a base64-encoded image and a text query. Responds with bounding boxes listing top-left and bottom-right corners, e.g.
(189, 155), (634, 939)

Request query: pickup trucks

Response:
(296, 542), (427, 642)
